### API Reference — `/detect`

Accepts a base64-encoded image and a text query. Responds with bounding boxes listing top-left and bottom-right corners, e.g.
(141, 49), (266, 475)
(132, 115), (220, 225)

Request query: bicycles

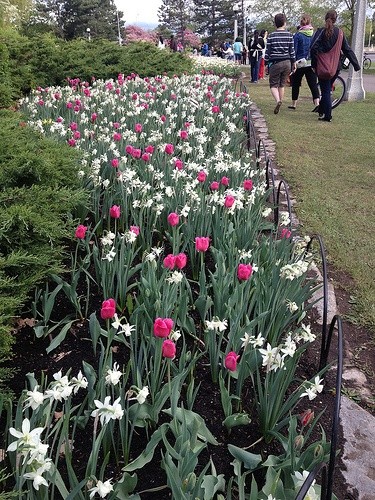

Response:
(316, 56), (351, 109)
(363, 51), (372, 71)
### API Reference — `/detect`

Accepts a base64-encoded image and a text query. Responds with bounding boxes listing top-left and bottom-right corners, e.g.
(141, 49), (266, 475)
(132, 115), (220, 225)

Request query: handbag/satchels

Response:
(317, 28), (343, 80)
(240, 47), (243, 52)
(296, 58), (307, 68)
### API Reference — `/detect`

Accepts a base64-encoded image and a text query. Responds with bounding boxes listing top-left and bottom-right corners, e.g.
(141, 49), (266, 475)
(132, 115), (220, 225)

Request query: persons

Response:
(288, 15), (321, 112)
(257, 29), (267, 80)
(310, 10), (361, 122)
(157, 35), (247, 65)
(264, 13), (297, 114)
(248, 30), (265, 83)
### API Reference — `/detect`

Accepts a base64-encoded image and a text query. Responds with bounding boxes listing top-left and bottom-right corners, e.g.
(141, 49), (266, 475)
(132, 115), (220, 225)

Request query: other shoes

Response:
(318, 116), (331, 122)
(249, 80), (257, 83)
(319, 110), (323, 117)
(273, 100), (282, 114)
(259, 77), (266, 81)
(288, 106), (296, 109)
(311, 105), (320, 112)
